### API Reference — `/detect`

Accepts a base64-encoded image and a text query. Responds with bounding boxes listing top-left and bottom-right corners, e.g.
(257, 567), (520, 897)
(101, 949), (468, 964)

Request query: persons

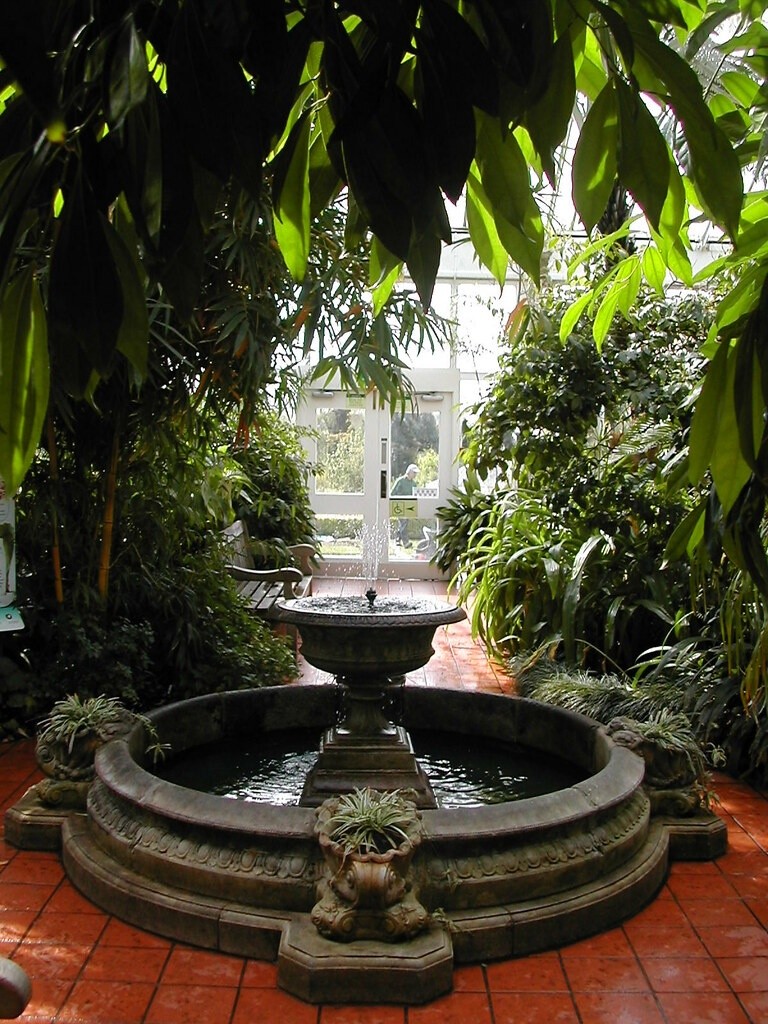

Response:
(390, 463), (420, 548)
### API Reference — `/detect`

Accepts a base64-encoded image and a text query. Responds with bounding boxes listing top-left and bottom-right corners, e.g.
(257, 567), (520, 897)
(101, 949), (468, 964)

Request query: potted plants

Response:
(310, 784), (433, 944)
(29, 690), (173, 807)
(606, 707), (727, 818)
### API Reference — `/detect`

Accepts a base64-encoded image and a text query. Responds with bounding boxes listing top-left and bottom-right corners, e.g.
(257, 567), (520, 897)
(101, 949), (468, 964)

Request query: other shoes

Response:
(404, 543), (412, 549)
(395, 540), (401, 546)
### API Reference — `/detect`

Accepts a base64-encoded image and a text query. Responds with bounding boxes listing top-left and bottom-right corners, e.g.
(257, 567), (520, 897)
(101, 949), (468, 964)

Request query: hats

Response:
(406, 464), (420, 474)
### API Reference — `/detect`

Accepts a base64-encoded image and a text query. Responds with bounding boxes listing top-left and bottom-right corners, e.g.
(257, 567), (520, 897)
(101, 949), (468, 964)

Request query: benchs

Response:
(214, 517), (315, 666)
